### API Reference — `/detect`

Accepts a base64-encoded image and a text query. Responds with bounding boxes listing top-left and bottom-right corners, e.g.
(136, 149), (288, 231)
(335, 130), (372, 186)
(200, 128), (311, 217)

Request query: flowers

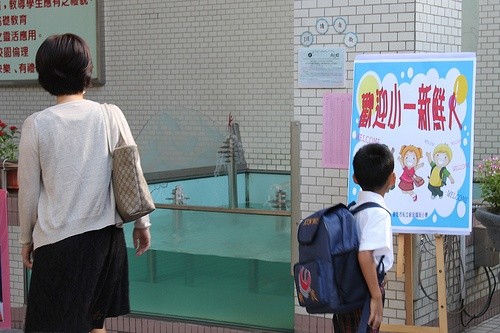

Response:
(473, 154), (500, 215)
(0, 120), (20, 162)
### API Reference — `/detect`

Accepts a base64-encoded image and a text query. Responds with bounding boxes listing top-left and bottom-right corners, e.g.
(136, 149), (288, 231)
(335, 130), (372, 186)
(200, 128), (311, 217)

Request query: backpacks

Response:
(294, 201), (391, 313)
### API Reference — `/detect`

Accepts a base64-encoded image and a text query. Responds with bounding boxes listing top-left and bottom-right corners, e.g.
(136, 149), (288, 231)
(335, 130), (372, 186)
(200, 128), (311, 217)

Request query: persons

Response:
(17, 33), (151, 333)
(333, 142), (397, 333)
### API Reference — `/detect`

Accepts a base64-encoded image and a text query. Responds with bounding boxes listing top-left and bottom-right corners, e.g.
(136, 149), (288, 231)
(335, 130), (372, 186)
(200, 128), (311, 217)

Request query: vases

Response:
(0, 160), (19, 192)
(475, 206), (500, 252)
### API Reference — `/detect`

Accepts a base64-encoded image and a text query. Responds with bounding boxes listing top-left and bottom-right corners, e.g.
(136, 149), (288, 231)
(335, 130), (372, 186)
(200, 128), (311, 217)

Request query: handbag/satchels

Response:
(100, 104), (156, 224)
